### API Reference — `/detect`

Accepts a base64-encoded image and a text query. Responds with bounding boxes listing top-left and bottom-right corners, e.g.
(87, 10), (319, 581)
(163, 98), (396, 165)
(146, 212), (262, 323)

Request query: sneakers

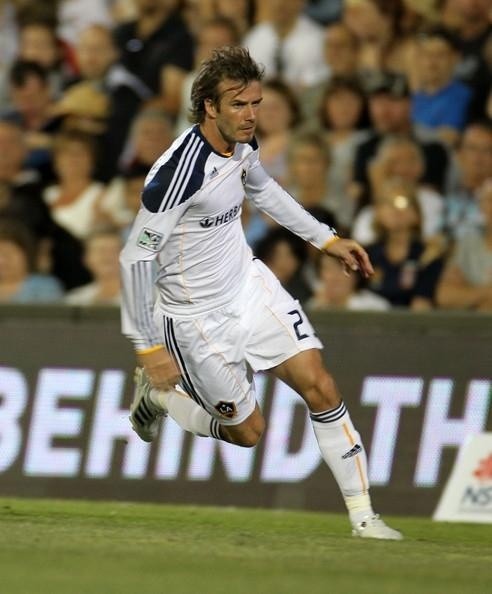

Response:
(129, 367), (167, 442)
(351, 514), (404, 539)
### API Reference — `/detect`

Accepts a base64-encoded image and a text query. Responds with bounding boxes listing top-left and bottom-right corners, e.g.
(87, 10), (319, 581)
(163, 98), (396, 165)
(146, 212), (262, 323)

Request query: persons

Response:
(115, 45), (406, 542)
(1, 1), (490, 322)
